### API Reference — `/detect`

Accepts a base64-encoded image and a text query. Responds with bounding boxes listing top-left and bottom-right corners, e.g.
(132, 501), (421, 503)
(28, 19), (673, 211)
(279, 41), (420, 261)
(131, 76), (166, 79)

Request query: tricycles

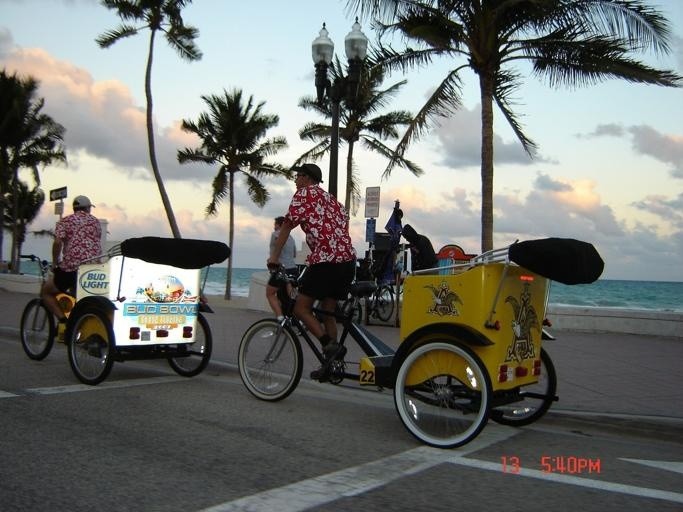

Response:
(17, 236), (232, 386)
(236, 236), (604, 449)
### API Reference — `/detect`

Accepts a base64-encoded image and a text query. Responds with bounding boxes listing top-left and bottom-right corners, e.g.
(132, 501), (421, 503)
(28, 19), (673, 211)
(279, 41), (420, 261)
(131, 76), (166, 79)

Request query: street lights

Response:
(311, 15), (369, 196)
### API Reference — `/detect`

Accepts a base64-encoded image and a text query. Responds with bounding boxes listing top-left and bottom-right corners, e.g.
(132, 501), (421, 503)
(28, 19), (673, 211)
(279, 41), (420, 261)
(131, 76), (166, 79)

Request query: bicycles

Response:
(341, 256), (395, 326)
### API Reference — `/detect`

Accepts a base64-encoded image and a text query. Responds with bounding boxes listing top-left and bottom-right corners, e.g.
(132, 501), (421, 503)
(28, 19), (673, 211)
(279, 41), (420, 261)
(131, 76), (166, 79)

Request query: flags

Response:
(382, 207), (403, 251)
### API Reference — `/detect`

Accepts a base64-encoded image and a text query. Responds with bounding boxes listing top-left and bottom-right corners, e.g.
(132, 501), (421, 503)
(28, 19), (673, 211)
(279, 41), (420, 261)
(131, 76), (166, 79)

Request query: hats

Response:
(290, 163), (323, 184)
(71, 194), (96, 210)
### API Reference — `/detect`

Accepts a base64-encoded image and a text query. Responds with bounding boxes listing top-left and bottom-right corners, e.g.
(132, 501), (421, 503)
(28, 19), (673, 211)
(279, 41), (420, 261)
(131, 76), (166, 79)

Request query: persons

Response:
(266, 215), (299, 337)
(42, 194), (102, 336)
(398, 223), (441, 277)
(266, 160), (357, 381)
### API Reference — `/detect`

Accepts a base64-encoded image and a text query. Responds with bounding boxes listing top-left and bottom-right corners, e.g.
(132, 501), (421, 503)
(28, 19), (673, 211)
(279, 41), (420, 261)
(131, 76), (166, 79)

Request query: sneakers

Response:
(310, 336), (350, 385)
